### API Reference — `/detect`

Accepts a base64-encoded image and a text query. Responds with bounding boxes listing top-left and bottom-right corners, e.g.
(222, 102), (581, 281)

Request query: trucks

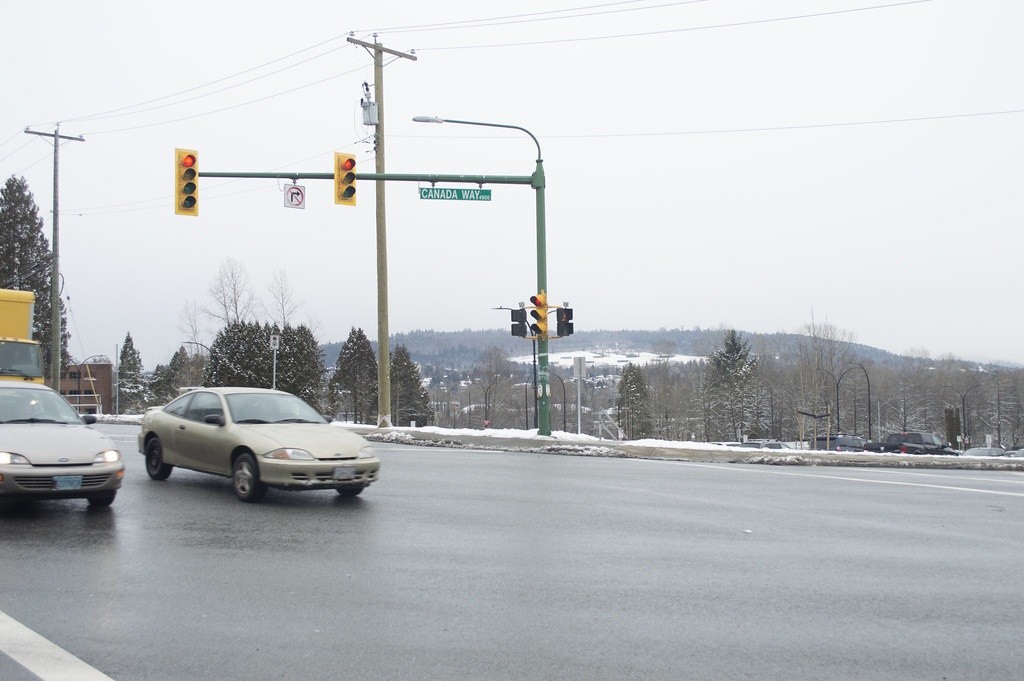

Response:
(0, 289), (46, 387)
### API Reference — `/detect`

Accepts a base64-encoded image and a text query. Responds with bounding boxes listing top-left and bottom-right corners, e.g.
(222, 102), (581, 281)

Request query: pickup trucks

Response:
(862, 432), (960, 457)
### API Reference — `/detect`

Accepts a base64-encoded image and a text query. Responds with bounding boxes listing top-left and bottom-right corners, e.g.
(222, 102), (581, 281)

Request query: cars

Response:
(811, 436), (866, 453)
(137, 388), (380, 505)
(738, 440), (792, 452)
(963, 448), (1008, 460)
(1006, 449), (1024, 458)
(1, 381), (126, 509)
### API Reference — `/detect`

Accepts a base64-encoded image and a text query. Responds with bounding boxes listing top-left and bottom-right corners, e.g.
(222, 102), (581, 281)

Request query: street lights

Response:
(412, 112), (552, 438)
(942, 383), (983, 452)
(815, 366), (860, 436)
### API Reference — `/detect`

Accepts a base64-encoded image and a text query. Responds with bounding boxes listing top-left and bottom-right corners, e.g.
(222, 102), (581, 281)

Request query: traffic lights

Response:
(510, 308), (528, 337)
(529, 292), (550, 334)
(173, 148), (202, 217)
(556, 308), (576, 337)
(334, 152), (358, 206)
